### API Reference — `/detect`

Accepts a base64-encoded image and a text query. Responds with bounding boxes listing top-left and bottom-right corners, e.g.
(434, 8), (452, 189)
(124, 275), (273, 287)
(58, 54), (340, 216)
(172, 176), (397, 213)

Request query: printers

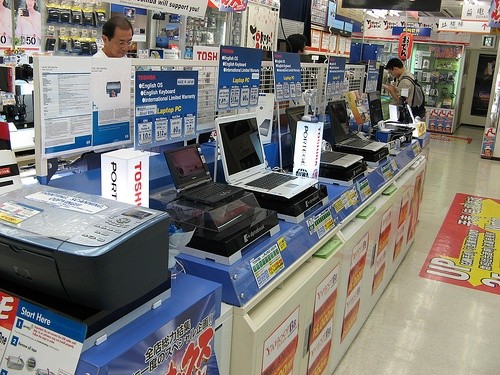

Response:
(0, 182), (169, 310)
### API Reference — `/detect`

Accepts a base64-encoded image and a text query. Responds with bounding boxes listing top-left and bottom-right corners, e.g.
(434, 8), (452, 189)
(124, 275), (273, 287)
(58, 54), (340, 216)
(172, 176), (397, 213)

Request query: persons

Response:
(285, 33), (306, 54)
(15, 0), (42, 48)
(384, 58), (426, 124)
(0, 0), (12, 47)
(92, 15), (134, 59)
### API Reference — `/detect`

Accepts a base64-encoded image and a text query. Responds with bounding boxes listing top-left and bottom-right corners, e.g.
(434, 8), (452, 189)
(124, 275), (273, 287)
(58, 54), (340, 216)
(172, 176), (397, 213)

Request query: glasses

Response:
(118, 41), (132, 47)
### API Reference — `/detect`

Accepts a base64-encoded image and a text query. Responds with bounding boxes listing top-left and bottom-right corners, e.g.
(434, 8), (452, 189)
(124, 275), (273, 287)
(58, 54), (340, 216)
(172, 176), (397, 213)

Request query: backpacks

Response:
(397, 76), (426, 119)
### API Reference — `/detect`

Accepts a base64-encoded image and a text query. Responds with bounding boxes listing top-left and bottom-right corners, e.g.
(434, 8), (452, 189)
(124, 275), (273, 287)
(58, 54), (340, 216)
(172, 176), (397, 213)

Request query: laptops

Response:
(164, 143), (244, 206)
(215, 91), (416, 199)
